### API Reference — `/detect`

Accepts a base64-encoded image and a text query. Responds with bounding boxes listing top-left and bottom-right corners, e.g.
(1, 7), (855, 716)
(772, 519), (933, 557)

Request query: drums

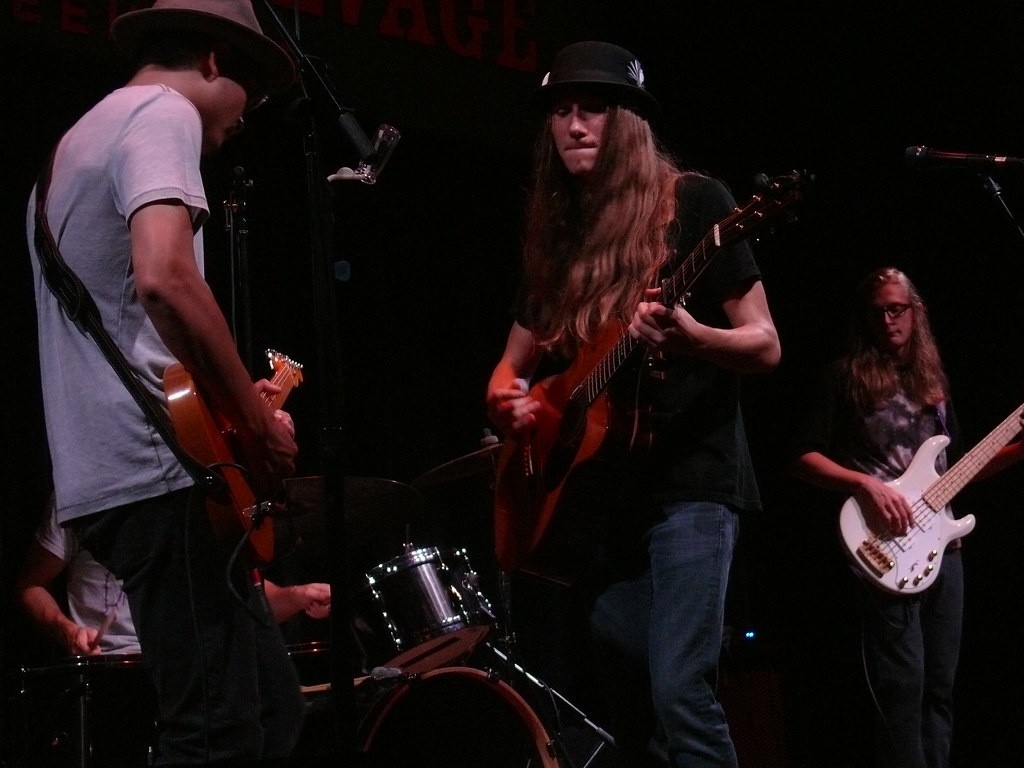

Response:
(52, 654), (150, 767)
(282, 666), (562, 768)
(342, 543), (497, 681)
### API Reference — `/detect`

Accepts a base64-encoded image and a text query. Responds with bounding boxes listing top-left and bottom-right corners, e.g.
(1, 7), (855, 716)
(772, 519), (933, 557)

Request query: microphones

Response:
(906, 145), (1024, 170)
(357, 123), (401, 184)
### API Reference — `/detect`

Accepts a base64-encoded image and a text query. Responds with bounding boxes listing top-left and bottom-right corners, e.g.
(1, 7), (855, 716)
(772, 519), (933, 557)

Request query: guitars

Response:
(162, 346), (309, 561)
(838, 402), (1023, 595)
(489, 164), (812, 575)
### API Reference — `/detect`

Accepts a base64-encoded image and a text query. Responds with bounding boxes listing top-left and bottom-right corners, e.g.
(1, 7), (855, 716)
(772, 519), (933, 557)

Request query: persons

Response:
(15, 0), (333, 768)
(787, 267), (1024, 768)
(485, 41), (782, 768)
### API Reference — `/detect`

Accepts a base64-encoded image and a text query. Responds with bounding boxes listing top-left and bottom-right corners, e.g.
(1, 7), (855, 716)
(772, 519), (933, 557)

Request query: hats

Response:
(541, 41), (659, 105)
(109, 0), (297, 92)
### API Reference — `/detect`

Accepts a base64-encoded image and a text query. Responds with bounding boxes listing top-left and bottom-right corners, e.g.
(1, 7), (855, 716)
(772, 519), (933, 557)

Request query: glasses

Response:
(867, 304), (912, 321)
(249, 80), (273, 112)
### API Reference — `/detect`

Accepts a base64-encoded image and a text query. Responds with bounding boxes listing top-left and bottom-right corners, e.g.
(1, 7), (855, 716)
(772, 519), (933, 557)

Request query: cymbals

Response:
(282, 474), (421, 536)
(407, 442), (505, 490)
